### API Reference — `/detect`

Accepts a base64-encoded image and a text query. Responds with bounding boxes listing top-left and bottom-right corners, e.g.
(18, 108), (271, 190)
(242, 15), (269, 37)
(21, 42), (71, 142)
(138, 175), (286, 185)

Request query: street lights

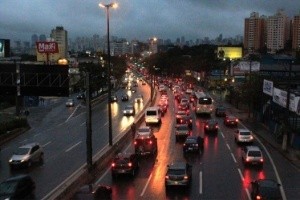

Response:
(98, 1), (119, 147)
(147, 37), (157, 101)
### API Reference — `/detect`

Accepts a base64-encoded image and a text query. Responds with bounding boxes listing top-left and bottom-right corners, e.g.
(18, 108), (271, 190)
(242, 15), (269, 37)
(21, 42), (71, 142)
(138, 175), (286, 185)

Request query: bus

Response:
(194, 91), (215, 115)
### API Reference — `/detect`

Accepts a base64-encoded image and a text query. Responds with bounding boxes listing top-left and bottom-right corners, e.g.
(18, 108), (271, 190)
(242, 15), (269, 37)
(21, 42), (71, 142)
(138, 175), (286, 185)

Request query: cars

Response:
(70, 134), (283, 200)
(110, 68), (146, 115)
(128, 60), (253, 144)
(0, 142), (45, 200)
(66, 93), (85, 107)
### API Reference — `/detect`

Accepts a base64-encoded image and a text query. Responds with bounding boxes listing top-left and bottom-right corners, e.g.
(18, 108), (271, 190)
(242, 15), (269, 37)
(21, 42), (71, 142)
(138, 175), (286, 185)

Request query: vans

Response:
(144, 106), (162, 125)
